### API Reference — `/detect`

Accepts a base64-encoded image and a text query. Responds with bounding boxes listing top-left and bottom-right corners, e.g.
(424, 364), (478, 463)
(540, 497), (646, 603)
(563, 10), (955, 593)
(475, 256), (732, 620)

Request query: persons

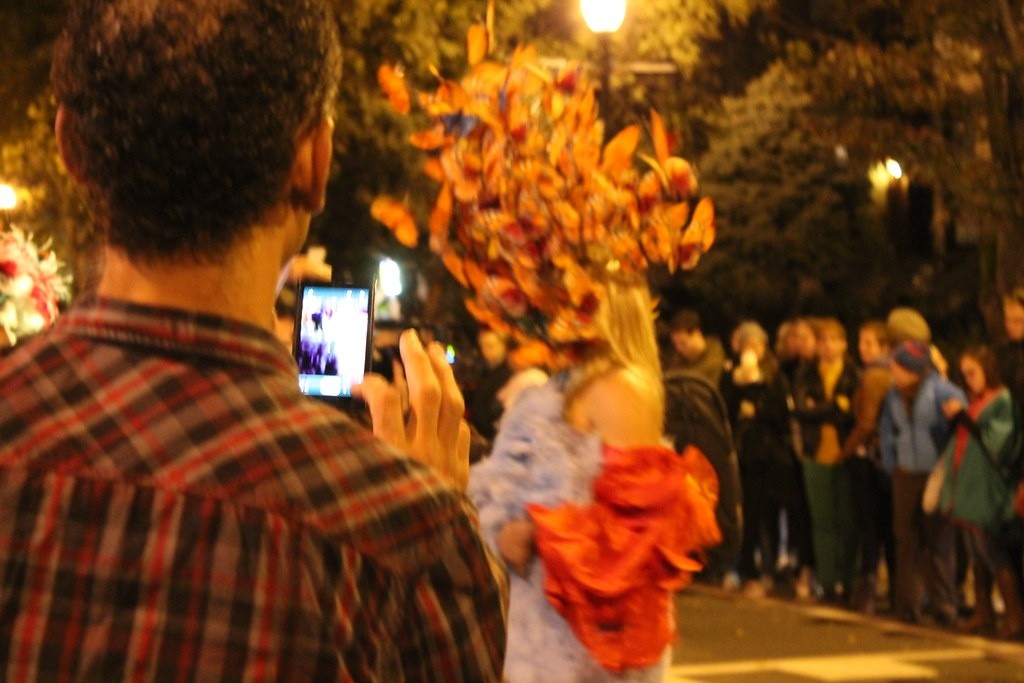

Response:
(651, 285), (1024, 639)
(0, 0), (674, 683)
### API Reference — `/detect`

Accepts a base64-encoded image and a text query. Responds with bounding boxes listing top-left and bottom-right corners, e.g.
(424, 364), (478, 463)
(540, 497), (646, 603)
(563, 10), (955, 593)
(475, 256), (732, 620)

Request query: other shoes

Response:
(723, 566), (1024, 641)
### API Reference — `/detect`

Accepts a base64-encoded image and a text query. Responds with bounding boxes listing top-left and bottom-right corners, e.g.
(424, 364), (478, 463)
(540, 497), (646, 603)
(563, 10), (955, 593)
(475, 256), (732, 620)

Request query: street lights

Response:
(577, 0), (630, 138)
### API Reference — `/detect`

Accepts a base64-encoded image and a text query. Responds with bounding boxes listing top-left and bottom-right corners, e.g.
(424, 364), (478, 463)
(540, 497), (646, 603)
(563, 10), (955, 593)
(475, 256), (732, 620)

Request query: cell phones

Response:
(286, 271), (376, 411)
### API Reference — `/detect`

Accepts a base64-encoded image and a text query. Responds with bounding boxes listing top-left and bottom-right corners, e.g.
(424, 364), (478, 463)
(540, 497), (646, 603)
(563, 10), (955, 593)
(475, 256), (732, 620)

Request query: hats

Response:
(895, 338), (930, 375)
